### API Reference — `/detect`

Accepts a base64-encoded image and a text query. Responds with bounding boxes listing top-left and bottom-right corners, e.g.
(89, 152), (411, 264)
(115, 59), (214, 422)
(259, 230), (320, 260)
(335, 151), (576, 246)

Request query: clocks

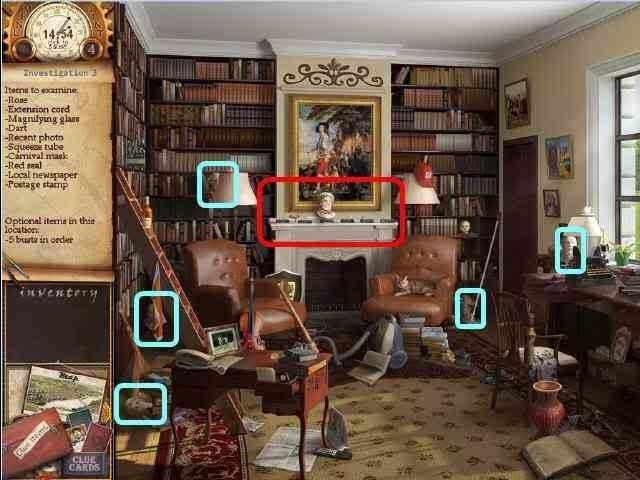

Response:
(1, 1), (113, 63)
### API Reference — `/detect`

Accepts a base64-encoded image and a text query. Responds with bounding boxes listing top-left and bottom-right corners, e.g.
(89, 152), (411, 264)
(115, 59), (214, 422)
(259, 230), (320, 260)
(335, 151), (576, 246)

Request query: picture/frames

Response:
(204, 323), (243, 358)
(504, 78), (529, 129)
(289, 94), (381, 212)
(545, 136), (574, 180)
(543, 190), (561, 218)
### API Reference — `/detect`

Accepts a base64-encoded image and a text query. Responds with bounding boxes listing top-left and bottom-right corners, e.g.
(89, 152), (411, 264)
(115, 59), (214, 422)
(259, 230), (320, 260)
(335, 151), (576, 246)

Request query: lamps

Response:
(563, 217), (603, 257)
(211, 172), (258, 241)
(394, 173), (441, 236)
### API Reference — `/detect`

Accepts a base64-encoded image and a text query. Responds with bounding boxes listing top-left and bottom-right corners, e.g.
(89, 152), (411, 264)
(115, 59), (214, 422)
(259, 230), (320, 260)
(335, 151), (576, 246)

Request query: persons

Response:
(561, 235), (579, 263)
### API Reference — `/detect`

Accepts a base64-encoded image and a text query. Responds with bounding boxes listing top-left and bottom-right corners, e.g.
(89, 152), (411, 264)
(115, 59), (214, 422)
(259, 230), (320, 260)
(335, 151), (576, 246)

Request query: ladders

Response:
(116, 167), (247, 436)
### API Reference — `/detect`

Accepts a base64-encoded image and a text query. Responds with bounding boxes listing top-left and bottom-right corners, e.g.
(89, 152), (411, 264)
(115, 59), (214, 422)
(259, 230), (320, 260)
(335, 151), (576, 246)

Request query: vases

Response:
(532, 381), (565, 435)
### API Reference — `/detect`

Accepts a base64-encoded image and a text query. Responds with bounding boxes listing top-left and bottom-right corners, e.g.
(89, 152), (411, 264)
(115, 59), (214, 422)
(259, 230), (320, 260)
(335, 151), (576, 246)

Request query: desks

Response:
(162, 351), (334, 480)
(517, 273), (640, 389)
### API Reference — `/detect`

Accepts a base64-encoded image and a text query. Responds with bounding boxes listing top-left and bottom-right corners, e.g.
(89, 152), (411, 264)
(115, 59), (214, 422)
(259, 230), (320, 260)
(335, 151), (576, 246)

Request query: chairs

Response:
(183, 240), (307, 339)
(489, 288), (583, 427)
(362, 235), (461, 326)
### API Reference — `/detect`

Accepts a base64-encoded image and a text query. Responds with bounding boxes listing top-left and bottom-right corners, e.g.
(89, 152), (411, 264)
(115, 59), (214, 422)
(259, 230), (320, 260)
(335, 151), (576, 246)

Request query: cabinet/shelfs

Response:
(392, 63), (500, 285)
(115, 1), (144, 392)
(145, 53), (277, 315)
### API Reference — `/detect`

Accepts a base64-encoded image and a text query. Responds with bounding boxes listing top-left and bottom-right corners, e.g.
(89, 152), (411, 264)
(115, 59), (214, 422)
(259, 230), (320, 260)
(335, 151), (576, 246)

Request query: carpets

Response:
(153, 328), (640, 480)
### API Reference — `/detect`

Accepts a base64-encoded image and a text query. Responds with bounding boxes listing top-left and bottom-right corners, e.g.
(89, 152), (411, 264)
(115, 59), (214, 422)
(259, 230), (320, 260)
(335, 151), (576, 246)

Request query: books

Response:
(581, 257), (640, 297)
(2, 365), (114, 480)
(118, 11), (499, 388)
(520, 428), (619, 479)
